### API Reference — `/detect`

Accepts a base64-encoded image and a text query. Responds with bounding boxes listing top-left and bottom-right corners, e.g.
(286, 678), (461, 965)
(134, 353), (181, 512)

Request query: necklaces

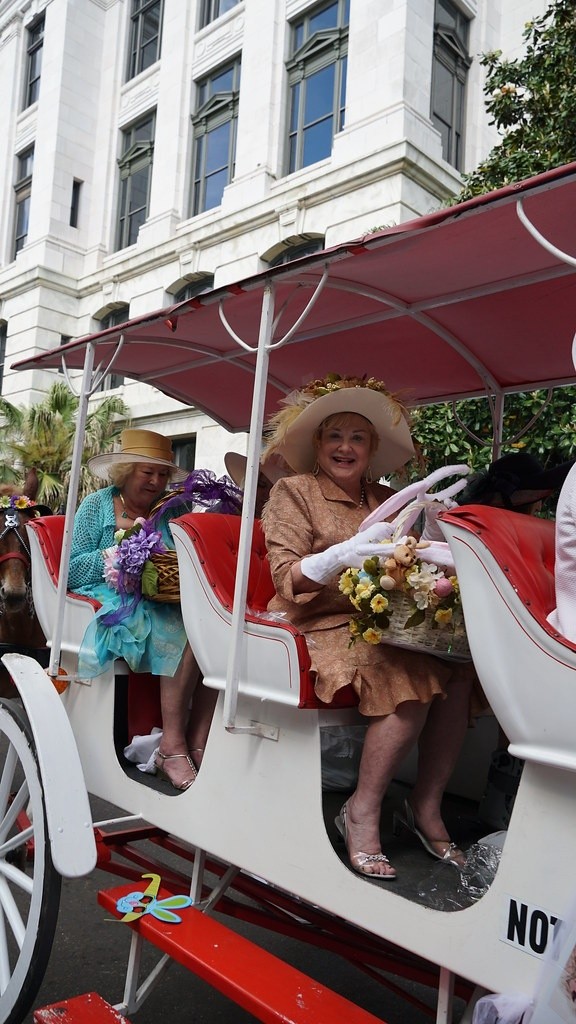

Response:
(120, 493), (137, 520)
(359, 484), (364, 508)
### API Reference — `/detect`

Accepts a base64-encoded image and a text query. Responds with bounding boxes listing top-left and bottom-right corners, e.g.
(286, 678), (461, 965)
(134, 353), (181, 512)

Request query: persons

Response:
(546, 459), (576, 644)
(456, 450), (576, 830)
(64, 429), (297, 788)
(224, 377), (478, 879)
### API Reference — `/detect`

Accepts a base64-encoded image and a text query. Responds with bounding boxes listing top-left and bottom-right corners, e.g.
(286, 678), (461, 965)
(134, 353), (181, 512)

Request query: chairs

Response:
(23, 508), (111, 658)
(431, 501), (576, 776)
(166, 510), (376, 713)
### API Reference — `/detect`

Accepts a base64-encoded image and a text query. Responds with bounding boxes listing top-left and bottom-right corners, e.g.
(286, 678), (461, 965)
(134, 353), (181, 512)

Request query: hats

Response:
(87, 428), (190, 484)
(224, 451), (289, 491)
(265, 373), (428, 482)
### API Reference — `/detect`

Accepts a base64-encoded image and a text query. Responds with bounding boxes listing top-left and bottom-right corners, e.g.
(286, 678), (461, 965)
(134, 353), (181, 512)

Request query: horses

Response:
(0, 467), (53, 698)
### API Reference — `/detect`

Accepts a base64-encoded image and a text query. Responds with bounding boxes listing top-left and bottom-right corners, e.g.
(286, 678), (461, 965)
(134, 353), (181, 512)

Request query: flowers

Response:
(0, 494), (41, 510)
(336, 506), (475, 658)
(99, 514), (172, 596)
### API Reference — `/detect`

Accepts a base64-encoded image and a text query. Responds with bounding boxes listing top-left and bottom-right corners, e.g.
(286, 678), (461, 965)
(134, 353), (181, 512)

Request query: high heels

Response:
(333, 797), (397, 879)
(188, 748), (204, 771)
(154, 751), (198, 789)
(391, 796), (463, 864)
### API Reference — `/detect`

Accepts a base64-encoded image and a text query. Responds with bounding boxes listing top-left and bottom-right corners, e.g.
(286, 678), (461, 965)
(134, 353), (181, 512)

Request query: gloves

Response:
(300, 520), (408, 586)
(419, 499), (460, 542)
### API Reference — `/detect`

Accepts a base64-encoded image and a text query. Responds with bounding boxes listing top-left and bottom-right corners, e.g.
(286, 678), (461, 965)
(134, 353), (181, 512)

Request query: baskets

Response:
(103, 487), (240, 604)
(339, 501), (473, 665)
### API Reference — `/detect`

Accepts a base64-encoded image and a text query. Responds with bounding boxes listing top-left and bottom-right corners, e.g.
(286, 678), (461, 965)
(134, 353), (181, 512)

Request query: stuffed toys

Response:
(380, 537), (431, 598)
